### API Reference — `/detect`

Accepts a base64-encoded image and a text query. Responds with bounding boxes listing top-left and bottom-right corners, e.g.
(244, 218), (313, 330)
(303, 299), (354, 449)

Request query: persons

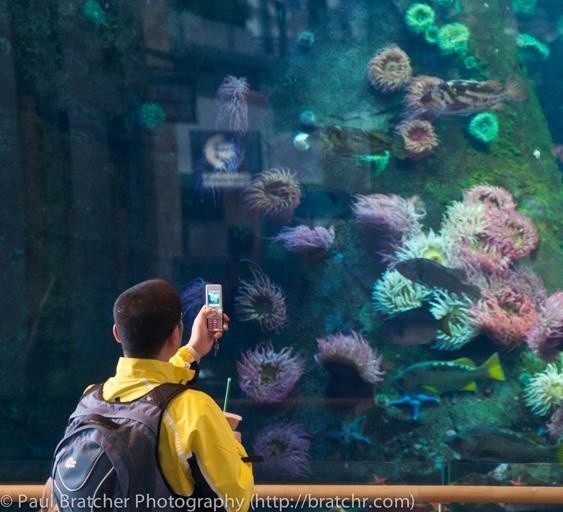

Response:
(40, 277), (256, 512)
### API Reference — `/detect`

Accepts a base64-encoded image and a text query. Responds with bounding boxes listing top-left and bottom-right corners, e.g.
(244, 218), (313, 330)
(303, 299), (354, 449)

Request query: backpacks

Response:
(53, 383), (207, 512)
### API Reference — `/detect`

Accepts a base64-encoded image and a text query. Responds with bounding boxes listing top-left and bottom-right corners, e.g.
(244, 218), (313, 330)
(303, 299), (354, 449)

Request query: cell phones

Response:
(205, 284), (223, 332)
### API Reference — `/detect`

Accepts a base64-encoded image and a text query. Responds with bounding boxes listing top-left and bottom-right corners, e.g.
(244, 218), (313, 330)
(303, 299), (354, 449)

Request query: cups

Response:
(216, 411), (242, 433)
